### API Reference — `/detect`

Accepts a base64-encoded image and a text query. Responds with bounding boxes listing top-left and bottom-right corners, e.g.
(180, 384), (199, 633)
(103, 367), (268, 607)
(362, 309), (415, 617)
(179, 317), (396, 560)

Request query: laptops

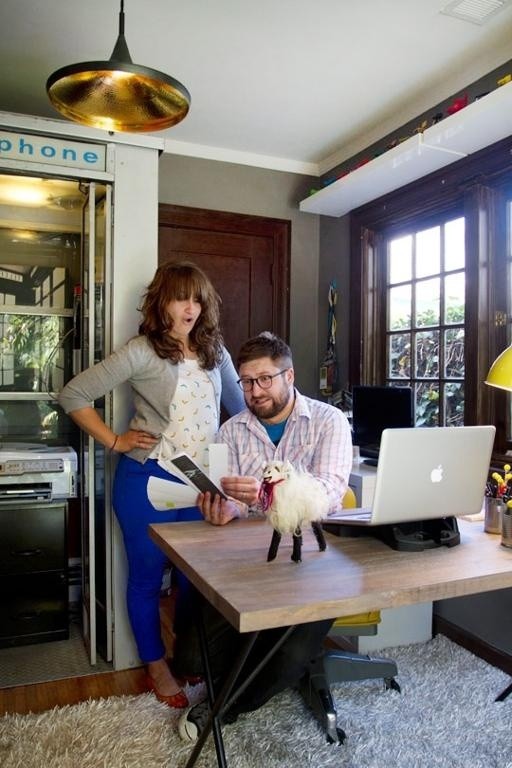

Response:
(321, 425), (496, 527)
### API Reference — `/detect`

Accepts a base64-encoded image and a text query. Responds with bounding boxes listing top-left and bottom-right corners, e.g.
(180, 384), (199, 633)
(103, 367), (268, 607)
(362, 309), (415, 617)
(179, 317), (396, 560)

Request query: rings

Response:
(242, 492), (247, 500)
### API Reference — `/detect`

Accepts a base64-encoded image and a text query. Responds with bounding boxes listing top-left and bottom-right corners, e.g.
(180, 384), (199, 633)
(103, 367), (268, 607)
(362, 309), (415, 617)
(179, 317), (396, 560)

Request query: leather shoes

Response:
(175, 663), (205, 685)
(145, 674), (189, 708)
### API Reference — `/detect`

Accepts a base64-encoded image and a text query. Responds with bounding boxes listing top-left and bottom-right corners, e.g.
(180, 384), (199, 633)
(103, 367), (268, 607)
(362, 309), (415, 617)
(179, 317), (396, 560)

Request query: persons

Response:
(59, 257), (250, 709)
(176, 332), (355, 742)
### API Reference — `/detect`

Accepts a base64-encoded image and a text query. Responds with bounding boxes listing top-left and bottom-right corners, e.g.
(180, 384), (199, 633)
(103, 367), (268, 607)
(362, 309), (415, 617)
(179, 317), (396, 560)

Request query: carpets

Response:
(1, 634), (511, 767)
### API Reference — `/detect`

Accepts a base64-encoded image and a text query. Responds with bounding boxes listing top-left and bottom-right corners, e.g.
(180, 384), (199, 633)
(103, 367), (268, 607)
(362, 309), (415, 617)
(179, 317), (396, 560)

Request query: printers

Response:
(0, 442), (78, 506)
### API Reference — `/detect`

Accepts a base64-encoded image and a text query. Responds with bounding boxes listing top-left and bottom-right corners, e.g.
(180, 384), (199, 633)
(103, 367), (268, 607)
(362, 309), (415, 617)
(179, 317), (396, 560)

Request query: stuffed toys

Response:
(257, 458), (334, 565)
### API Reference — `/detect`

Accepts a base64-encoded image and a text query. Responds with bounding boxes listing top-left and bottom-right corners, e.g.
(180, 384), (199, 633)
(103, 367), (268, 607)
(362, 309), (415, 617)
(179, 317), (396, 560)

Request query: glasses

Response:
(237, 369), (289, 392)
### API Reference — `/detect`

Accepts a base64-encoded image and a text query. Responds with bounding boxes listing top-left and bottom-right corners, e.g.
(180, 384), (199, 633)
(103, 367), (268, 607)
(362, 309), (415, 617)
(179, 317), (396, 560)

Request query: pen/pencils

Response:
(502, 478), (512, 514)
(485, 465), (512, 497)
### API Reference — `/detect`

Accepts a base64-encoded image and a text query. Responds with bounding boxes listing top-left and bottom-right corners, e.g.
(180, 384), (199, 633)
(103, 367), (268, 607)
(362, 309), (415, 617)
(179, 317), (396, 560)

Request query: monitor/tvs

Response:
(353, 386), (414, 445)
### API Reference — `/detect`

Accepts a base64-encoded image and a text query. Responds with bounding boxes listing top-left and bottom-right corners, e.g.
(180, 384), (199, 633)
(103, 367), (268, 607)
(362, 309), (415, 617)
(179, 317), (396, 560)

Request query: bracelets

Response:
(110, 433), (119, 452)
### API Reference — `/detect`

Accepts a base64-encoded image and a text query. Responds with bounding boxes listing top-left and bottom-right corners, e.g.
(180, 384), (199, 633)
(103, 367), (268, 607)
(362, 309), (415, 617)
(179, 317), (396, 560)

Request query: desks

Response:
(150, 506), (511, 768)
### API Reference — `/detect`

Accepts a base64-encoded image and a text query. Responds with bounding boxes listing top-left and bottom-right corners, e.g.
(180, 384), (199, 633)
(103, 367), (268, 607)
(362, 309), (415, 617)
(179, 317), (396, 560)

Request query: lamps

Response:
(46, 2), (190, 135)
(484, 341), (512, 391)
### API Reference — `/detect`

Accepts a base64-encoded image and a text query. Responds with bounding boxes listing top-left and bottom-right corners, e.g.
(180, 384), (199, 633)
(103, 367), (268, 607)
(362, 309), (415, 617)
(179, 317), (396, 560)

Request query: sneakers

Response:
(178, 698), (239, 742)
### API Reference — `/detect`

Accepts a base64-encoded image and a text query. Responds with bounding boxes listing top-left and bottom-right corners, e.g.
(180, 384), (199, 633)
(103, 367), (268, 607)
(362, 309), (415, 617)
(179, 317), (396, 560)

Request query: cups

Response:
(484, 498), (504, 534)
(501, 515), (512, 548)
